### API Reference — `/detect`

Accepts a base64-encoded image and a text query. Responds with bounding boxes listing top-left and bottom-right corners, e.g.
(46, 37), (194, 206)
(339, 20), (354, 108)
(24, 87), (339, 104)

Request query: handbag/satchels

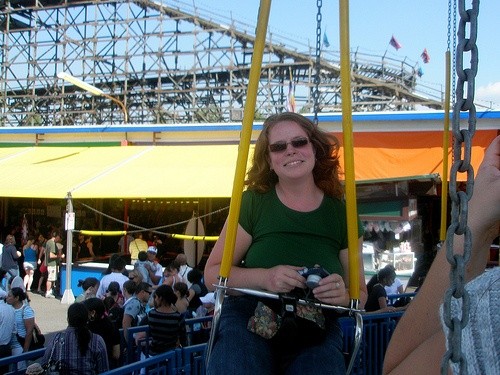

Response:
(46, 332), (64, 375)
(108, 298), (142, 327)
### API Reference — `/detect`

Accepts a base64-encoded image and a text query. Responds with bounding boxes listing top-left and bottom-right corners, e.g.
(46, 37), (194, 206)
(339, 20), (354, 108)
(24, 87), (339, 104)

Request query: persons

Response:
(139, 285), (195, 375)
(75, 227), (216, 375)
(0, 222), (95, 375)
(7, 287), (45, 361)
(382, 135), (500, 375)
(82, 297), (123, 369)
(364, 267), (413, 311)
(203, 111), (368, 375)
(384, 264), (404, 305)
(41, 302), (109, 375)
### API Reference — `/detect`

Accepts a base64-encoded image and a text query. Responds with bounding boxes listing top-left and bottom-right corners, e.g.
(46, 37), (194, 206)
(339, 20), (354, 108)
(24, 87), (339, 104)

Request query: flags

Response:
(323, 32), (330, 47)
(420, 48), (431, 64)
(416, 67), (424, 77)
(389, 35), (402, 51)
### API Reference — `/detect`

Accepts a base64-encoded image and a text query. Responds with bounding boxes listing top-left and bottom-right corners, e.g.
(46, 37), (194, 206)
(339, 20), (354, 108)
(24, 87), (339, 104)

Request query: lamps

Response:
(56, 72), (128, 125)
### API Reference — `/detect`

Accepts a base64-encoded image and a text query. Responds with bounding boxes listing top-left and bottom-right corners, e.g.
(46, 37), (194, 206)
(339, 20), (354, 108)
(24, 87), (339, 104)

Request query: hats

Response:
(147, 247), (158, 254)
(26, 363), (44, 374)
(200, 293), (215, 304)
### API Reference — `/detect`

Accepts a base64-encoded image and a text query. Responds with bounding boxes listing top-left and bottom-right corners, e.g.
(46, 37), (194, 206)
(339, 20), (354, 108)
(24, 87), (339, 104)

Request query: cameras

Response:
(298, 267), (331, 289)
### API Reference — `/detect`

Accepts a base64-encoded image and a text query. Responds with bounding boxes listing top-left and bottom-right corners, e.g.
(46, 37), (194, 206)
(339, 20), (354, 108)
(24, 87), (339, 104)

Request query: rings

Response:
(336, 282), (339, 288)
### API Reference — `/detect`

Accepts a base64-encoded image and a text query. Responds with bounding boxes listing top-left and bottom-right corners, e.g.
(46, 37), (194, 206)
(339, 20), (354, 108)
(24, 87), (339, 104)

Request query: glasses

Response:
(268, 137), (309, 152)
(144, 290), (152, 295)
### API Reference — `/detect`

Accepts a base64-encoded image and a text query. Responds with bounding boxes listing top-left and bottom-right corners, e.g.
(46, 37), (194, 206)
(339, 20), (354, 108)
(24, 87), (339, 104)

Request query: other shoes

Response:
(45, 293), (56, 298)
